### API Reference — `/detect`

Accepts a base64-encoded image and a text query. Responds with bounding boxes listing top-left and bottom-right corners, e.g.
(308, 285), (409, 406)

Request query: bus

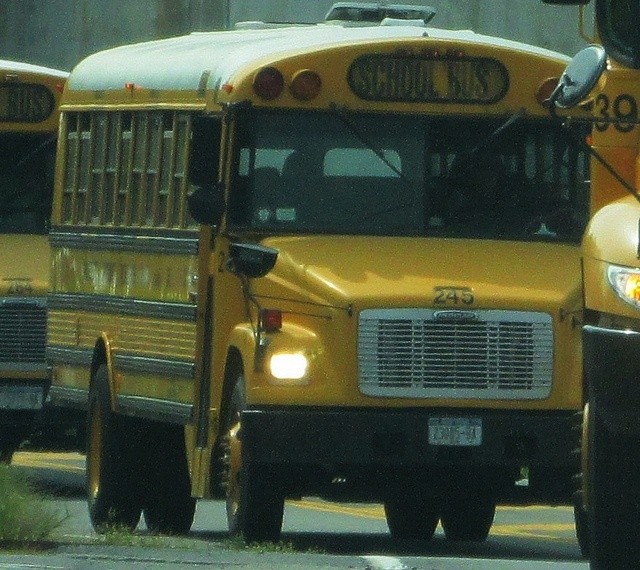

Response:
(0, 56), (69, 466)
(541, 0), (640, 570)
(37, 0), (589, 560)
